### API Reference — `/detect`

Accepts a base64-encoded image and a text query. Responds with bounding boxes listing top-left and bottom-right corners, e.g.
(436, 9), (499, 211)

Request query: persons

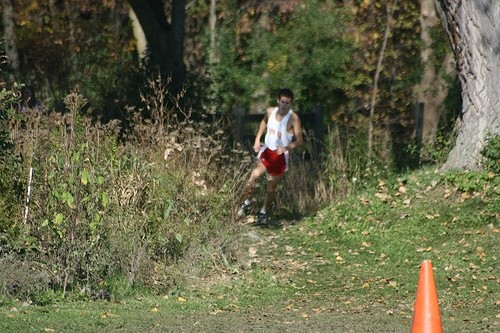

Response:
(236, 88), (303, 225)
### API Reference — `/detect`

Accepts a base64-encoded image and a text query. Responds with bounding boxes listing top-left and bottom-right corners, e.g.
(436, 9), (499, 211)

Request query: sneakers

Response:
(236, 199), (253, 219)
(259, 212), (267, 225)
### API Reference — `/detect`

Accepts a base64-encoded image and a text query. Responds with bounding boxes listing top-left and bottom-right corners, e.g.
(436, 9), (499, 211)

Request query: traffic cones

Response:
(409, 258), (446, 333)
